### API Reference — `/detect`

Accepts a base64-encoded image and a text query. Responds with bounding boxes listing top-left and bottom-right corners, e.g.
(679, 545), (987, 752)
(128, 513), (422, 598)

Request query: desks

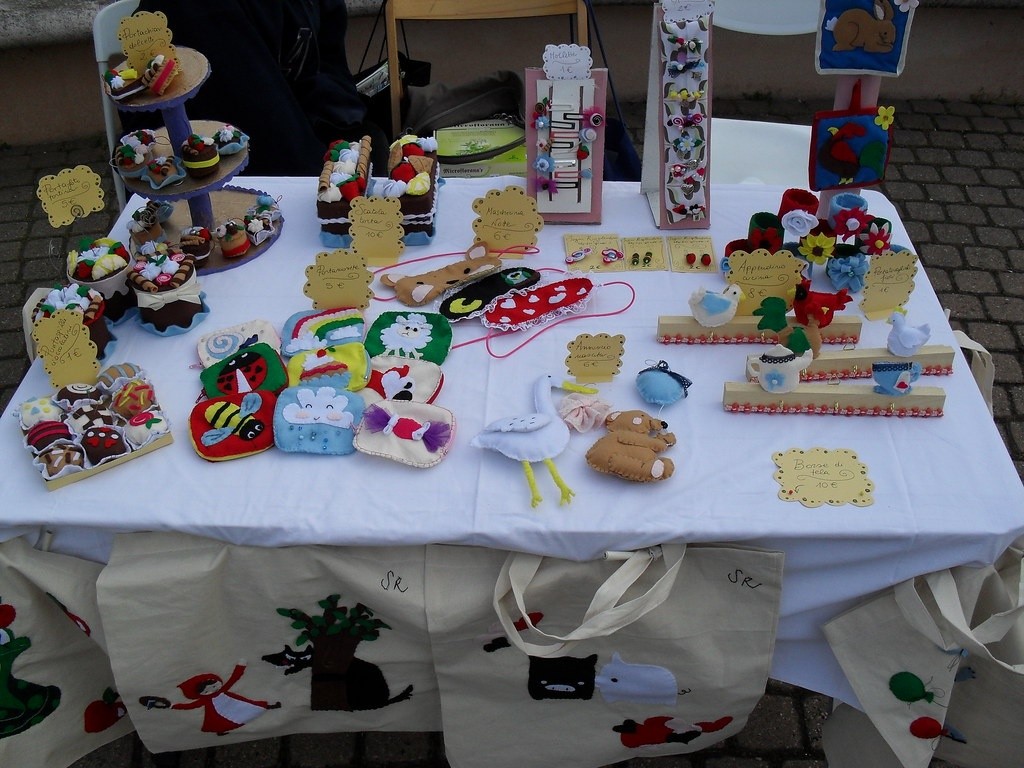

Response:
(2, 177), (1023, 767)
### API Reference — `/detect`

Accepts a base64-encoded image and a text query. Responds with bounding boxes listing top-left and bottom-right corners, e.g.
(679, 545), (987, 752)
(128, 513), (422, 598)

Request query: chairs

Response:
(93, 0), (139, 217)
(385, 0), (590, 142)
(712, 0), (820, 180)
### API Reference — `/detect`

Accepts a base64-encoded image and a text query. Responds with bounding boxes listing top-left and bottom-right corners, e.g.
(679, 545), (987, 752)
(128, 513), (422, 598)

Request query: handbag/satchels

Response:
(1, 532), (139, 768)
(423, 539), (790, 768)
(819, 536), (1024, 768)
(92, 529), (510, 754)
